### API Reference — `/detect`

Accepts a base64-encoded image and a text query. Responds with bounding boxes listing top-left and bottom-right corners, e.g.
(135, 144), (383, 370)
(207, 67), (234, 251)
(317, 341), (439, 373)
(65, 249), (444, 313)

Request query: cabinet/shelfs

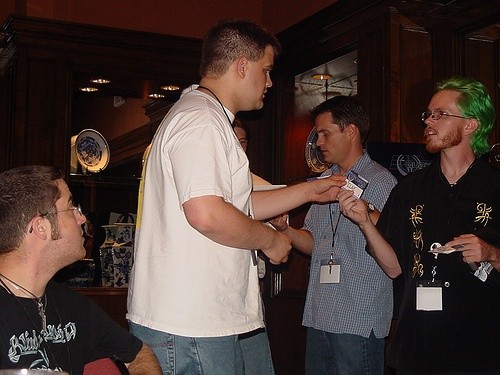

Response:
(63, 172), (140, 296)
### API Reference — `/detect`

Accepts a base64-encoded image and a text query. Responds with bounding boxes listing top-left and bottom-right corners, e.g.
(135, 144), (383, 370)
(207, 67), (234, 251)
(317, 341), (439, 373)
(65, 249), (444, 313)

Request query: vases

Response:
(112, 222), (135, 288)
(98, 225), (116, 287)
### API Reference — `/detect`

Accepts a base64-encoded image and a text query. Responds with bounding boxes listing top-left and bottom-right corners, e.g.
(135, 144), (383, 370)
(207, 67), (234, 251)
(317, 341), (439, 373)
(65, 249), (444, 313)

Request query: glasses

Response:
(422, 110), (471, 121)
(28, 201), (82, 233)
(240, 140), (248, 146)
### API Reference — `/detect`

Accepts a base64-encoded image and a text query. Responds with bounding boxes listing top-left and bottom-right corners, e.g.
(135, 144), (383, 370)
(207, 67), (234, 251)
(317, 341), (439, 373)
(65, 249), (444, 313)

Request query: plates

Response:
(75, 129), (110, 172)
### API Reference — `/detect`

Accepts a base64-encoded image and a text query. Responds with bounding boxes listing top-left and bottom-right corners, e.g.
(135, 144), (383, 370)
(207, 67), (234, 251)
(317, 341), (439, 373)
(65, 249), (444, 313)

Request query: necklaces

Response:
(0, 272), (72, 375)
(196, 85), (239, 140)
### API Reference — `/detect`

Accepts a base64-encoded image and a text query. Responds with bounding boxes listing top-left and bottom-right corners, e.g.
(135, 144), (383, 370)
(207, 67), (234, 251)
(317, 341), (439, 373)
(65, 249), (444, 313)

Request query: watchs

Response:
(366, 203), (375, 215)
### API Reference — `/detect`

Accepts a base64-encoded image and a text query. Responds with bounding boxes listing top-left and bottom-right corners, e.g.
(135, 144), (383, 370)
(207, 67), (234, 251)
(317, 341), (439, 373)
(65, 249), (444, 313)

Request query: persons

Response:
(125, 22), (347, 375)
(231, 118), (272, 279)
(266, 95), (399, 375)
(337, 78), (499, 375)
(0, 165), (161, 375)
